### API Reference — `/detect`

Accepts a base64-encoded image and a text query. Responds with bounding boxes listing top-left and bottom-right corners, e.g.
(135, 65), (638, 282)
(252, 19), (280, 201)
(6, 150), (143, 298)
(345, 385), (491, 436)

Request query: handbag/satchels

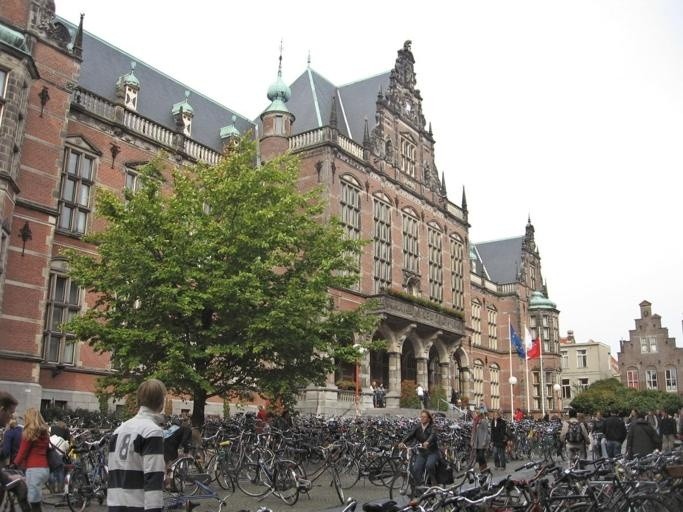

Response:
(48, 447), (63, 472)
(436, 457), (454, 484)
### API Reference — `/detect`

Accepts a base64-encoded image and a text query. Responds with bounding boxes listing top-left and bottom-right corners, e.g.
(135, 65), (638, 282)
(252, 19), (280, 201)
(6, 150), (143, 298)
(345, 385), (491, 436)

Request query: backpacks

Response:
(565, 420), (584, 443)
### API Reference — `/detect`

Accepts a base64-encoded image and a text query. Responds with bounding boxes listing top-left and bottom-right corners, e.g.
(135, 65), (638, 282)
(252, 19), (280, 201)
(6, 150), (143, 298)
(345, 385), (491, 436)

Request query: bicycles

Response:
(389, 442), (455, 506)
(0, 413), (683, 512)
(169, 426), (238, 498)
(234, 432), (300, 506)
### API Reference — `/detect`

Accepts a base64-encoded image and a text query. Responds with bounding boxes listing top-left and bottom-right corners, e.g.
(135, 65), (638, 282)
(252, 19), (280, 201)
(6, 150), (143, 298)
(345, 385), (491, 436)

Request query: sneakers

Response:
(495, 466), (505, 470)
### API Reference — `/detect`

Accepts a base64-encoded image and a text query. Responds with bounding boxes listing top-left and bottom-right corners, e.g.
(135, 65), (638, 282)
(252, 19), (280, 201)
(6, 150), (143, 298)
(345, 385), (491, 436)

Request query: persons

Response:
(487, 409), (509, 468)
(0, 406), (50, 511)
(658, 412), (677, 455)
(469, 405), (490, 471)
(0, 389), (26, 491)
(642, 410), (658, 433)
(1, 421), (28, 459)
(598, 408), (627, 461)
(559, 409), (591, 462)
(396, 410), (442, 491)
(622, 412), (662, 460)
(105, 378), (168, 512)
(162, 426), (201, 493)
(415, 383), (425, 403)
(254, 404), (267, 435)
(377, 383), (386, 407)
(513, 407), (524, 425)
(41, 418), (70, 494)
(369, 381), (381, 408)
(624, 408), (640, 430)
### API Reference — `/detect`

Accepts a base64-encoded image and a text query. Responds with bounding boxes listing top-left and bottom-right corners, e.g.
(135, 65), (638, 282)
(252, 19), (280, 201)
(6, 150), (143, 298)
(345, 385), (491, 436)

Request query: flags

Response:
(510, 323), (526, 361)
(520, 326), (532, 354)
(526, 334), (541, 359)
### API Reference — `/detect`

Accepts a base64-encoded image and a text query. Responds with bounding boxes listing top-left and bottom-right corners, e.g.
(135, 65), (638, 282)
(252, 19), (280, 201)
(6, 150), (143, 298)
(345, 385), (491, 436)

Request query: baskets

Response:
(665, 465), (683, 478)
